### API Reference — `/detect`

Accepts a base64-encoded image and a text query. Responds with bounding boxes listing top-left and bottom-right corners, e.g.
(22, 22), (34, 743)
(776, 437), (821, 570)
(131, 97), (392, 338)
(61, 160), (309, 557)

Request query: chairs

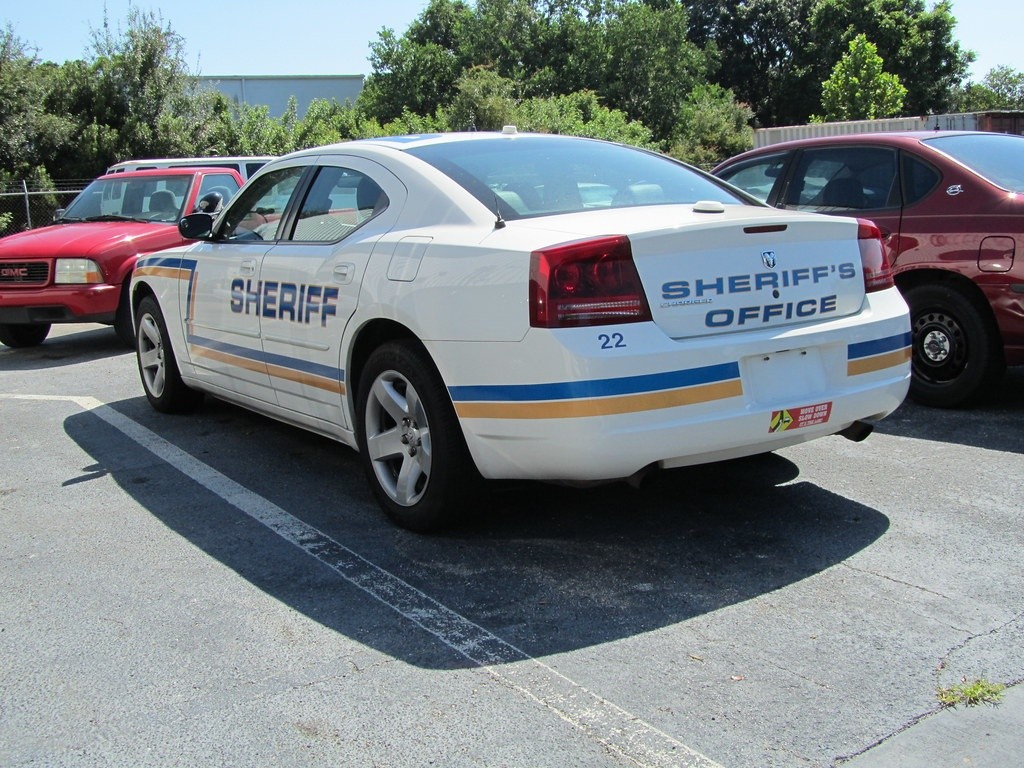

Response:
(148, 191), (180, 213)
(823, 179), (865, 209)
(205, 186), (232, 208)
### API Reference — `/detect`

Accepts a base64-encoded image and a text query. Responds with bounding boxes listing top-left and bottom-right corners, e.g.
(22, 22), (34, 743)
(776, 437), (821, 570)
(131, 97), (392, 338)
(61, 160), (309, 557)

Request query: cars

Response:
(0, 167), (257, 348)
(128, 124), (912, 533)
(707, 130), (1024, 409)
(521, 182), (619, 209)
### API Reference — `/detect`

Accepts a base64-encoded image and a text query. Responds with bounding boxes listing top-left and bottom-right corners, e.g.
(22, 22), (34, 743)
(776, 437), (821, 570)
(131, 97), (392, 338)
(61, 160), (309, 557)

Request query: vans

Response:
(101, 156), (281, 214)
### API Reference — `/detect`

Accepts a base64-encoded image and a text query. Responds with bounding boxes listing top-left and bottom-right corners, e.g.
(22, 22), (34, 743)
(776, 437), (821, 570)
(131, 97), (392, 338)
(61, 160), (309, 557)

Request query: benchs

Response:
(497, 184), (666, 215)
